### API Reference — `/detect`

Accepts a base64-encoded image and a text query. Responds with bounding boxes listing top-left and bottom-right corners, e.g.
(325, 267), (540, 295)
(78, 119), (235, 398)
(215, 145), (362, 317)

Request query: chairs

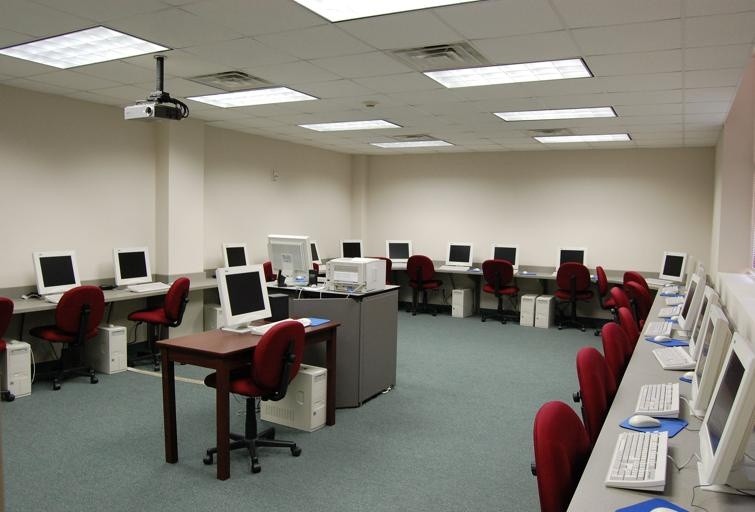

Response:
(625, 271), (649, 284)
(126, 277), (190, 373)
(573, 345), (611, 438)
(28, 284), (106, 391)
(620, 307), (641, 346)
(531, 400), (595, 512)
(0, 296), (15, 402)
(312, 264), (319, 277)
(602, 320), (633, 386)
(263, 261), (276, 282)
(407, 255), (445, 316)
(611, 287), (635, 327)
(553, 263), (593, 334)
(203, 319), (306, 473)
(630, 285), (653, 331)
(363, 257), (393, 285)
(480, 260), (520, 324)
(597, 264), (634, 332)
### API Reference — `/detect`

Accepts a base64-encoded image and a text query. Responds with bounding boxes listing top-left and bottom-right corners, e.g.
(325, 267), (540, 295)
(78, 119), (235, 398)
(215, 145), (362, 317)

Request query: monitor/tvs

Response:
(689, 286), (720, 359)
(216, 263), (272, 333)
(660, 250), (688, 283)
(268, 234), (313, 287)
(112, 246), (152, 286)
(677, 274), (704, 336)
(311, 240), (321, 263)
(445, 243), (473, 266)
(689, 304), (733, 415)
(223, 243), (252, 267)
(697, 332), (755, 495)
(556, 247), (587, 270)
(32, 250), (81, 295)
(386, 240), (412, 263)
(340, 240), (363, 258)
(492, 245), (519, 269)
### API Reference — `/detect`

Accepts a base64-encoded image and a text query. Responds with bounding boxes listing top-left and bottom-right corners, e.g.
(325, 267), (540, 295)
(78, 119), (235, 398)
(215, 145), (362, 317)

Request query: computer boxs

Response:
(535, 295), (554, 328)
(452, 289), (472, 318)
(90, 324), (127, 374)
(5, 339), (32, 399)
(261, 362), (327, 433)
(204, 303), (223, 331)
(520, 293), (537, 326)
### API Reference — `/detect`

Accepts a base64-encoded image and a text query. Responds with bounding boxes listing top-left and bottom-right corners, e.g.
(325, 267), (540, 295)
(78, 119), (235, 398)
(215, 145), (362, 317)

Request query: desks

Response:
(155, 316), (343, 482)
(265, 276), (400, 410)
(565, 286), (755, 512)
(390, 258), (686, 320)
(0, 269), (221, 365)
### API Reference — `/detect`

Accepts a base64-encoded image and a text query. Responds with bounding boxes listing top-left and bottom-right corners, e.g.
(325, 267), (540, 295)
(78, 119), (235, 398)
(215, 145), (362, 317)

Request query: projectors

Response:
(123, 90), (187, 120)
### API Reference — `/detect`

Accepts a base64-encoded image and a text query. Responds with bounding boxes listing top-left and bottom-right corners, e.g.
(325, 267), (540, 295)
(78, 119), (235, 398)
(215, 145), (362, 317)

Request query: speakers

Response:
(264, 293), (289, 321)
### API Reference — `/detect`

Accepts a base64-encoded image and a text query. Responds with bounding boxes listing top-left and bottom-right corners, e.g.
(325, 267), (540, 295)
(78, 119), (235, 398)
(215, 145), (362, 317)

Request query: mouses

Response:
(684, 371), (695, 379)
(671, 316), (678, 320)
(654, 336), (672, 342)
(630, 416), (662, 427)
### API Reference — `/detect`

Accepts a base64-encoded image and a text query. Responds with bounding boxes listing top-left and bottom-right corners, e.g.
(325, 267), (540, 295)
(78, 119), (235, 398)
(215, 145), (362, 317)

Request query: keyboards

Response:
(436, 265), (469, 272)
(251, 318), (311, 335)
(645, 277), (673, 286)
(658, 307), (681, 317)
(645, 320), (672, 337)
(660, 286), (679, 294)
(635, 383), (680, 419)
(666, 297), (683, 305)
(653, 347), (696, 370)
(128, 282), (170, 292)
(604, 432), (668, 494)
(45, 294), (64, 303)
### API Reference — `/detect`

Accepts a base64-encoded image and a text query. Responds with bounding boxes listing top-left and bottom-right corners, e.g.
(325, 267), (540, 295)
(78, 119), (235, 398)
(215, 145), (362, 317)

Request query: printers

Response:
(327, 257), (386, 293)
